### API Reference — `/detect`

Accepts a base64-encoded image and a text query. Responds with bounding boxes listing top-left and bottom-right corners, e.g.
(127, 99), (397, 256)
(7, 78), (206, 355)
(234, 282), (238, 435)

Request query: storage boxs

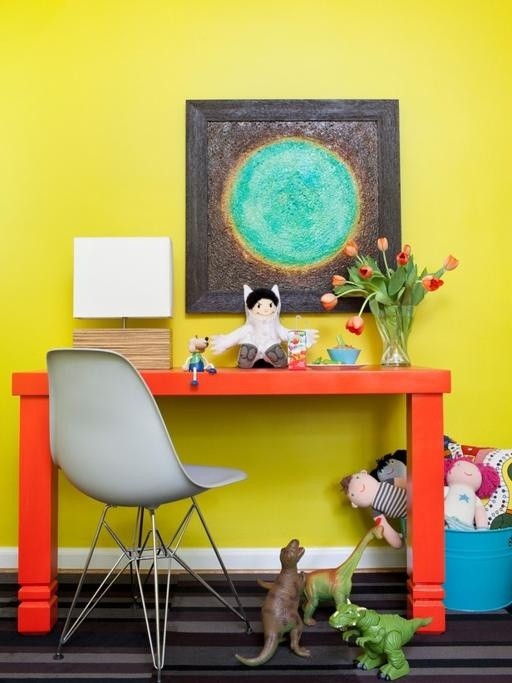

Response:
(401, 519), (512, 613)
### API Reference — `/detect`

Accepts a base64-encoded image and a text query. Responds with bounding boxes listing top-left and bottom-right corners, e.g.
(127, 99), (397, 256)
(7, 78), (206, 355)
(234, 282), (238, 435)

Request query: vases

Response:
(376, 304), (418, 369)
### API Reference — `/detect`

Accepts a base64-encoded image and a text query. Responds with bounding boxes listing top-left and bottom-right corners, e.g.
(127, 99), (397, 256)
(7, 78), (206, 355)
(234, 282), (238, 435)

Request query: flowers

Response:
(319, 236), (459, 335)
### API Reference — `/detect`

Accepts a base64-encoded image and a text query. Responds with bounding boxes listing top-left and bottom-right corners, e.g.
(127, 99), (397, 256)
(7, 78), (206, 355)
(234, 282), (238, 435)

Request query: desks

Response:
(12, 365), (451, 635)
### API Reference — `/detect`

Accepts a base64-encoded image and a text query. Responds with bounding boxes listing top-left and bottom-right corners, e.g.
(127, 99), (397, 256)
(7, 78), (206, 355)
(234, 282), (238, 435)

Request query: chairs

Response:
(46, 348), (256, 682)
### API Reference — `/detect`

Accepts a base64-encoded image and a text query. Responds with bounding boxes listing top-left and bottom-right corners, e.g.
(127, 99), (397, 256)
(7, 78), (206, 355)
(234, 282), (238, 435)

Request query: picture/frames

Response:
(186, 101), (401, 315)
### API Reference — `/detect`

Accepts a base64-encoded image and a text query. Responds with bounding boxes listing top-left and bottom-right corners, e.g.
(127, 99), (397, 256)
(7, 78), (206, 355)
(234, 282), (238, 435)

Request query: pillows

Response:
(443, 436), (512, 530)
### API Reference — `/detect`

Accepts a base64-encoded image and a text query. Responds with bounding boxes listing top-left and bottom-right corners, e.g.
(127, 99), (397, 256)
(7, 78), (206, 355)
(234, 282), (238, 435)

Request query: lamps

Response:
(73, 236), (173, 370)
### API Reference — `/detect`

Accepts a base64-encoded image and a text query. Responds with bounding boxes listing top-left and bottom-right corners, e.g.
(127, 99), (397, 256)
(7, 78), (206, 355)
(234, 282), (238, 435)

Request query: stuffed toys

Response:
(210, 282), (319, 371)
(441, 456), (499, 531)
(342, 468), (407, 547)
(370, 449), (408, 480)
